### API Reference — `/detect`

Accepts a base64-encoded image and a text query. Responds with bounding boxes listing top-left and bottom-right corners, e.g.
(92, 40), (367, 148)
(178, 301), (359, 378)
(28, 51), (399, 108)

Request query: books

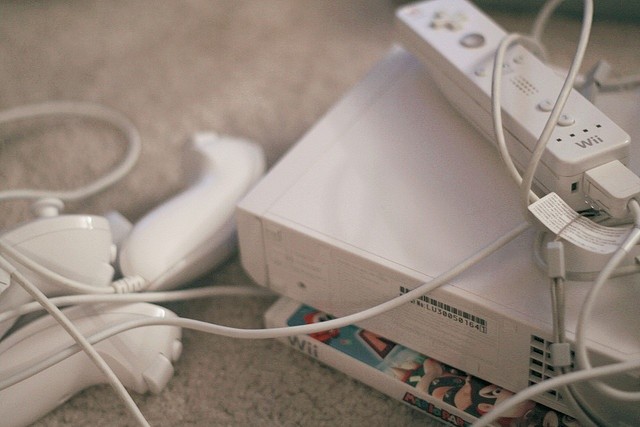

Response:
(261, 296), (592, 427)
